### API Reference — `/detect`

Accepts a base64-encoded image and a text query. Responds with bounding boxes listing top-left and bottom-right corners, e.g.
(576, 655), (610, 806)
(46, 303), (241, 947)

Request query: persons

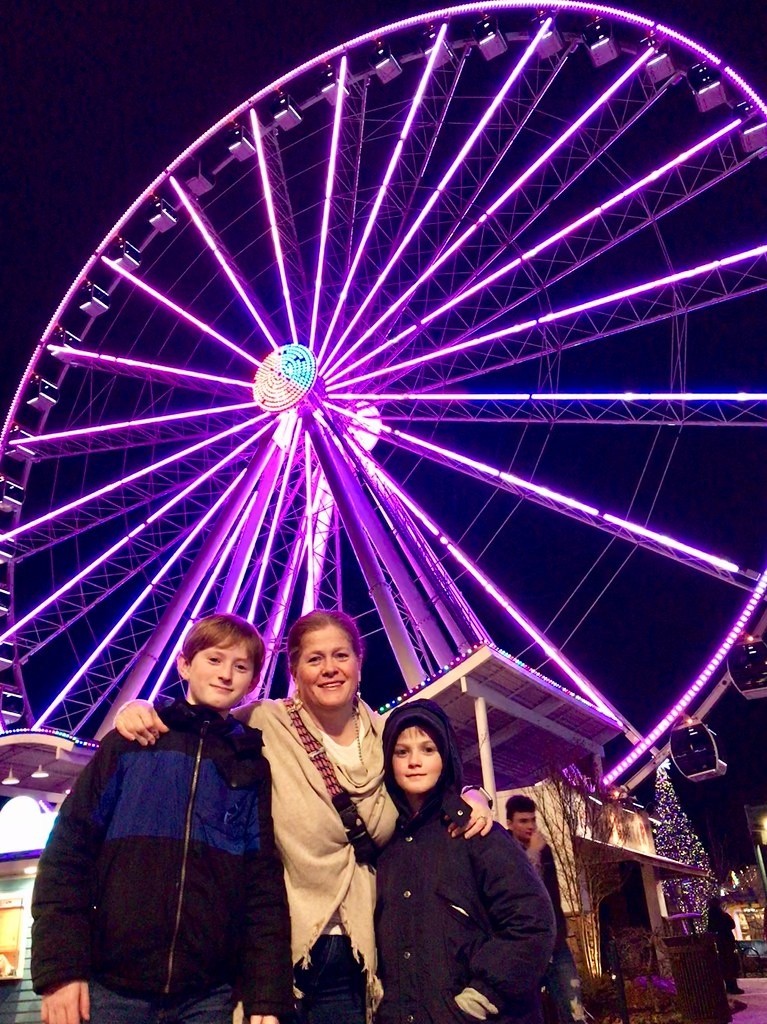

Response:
(708, 898), (745, 994)
(504, 794), (586, 1024)
(377, 699), (555, 1024)
(31, 616), (294, 1024)
(114, 612), (493, 1024)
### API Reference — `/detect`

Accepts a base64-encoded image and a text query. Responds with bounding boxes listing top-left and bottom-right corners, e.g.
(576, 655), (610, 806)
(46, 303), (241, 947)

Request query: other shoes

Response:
(726, 987), (744, 995)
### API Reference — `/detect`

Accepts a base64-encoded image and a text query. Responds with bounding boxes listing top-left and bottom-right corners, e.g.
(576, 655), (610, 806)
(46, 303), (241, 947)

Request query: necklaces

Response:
(353, 710), (365, 765)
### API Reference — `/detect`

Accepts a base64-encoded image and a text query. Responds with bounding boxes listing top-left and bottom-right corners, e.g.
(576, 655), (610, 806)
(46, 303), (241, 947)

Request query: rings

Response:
(478, 816), (487, 824)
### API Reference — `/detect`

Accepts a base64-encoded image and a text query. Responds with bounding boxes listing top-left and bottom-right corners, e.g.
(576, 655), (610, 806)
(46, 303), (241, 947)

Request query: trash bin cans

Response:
(661, 913), (733, 1024)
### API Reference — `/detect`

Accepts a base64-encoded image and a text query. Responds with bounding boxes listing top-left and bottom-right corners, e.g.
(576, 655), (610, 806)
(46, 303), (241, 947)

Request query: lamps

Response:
(1, 766), (21, 785)
(31, 763), (50, 778)
